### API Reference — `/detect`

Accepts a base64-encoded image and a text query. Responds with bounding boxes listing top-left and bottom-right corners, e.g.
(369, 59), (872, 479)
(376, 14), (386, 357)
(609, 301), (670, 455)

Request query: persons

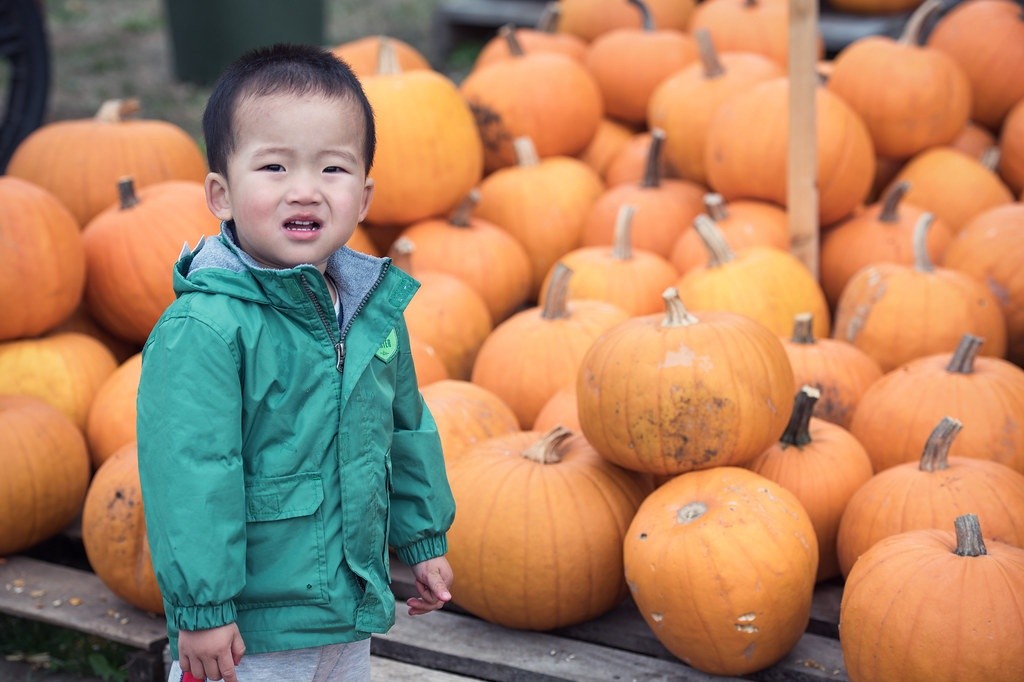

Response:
(135, 47), (456, 682)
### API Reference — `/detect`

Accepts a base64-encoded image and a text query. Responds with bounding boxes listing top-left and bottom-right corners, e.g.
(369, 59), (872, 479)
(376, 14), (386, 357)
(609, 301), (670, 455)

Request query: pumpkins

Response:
(0, 0), (1024, 682)
